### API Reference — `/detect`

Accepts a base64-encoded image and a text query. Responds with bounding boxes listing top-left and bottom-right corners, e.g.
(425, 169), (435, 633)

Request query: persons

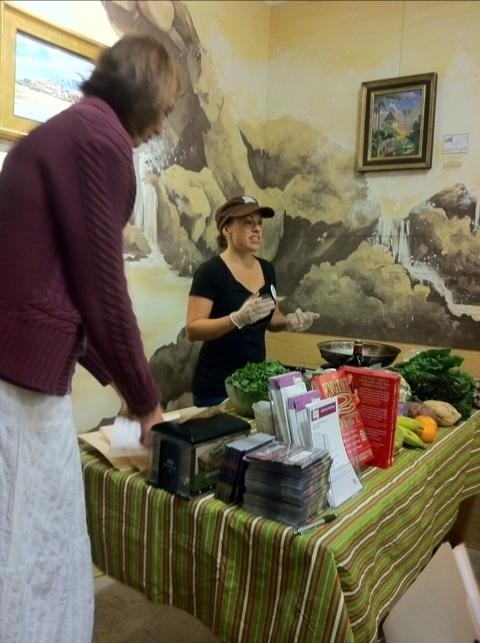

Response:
(184, 195), (320, 412)
(0, 34), (180, 642)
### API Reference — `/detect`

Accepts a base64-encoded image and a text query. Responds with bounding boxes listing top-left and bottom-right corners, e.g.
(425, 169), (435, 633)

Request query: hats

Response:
(215, 195), (275, 232)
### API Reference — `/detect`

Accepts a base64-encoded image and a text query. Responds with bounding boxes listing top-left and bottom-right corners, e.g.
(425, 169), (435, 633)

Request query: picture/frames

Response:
(353, 70), (437, 175)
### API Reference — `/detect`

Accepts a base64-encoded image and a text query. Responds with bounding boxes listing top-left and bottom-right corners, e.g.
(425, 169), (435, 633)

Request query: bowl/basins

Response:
(224, 378), (269, 419)
(316, 339), (401, 367)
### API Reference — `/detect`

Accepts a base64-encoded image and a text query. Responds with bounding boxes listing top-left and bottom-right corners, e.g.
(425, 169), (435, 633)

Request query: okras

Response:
(393, 416), (426, 453)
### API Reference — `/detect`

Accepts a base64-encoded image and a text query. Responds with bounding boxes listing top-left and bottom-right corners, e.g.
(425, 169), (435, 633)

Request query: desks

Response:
(66, 329), (480, 643)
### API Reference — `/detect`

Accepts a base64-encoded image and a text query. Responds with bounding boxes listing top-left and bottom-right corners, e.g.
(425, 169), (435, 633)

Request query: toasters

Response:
(148, 410), (252, 500)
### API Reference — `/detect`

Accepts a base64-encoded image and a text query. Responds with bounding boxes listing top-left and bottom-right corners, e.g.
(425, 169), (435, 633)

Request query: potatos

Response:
(407, 399), (461, 427)
